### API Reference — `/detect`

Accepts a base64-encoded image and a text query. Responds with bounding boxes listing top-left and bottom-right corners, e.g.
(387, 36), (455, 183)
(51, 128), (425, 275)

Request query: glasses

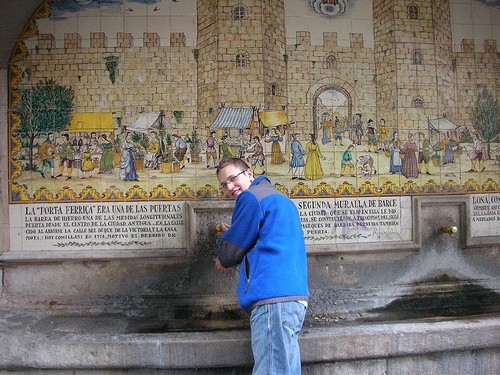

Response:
(221, 169), (248, 190)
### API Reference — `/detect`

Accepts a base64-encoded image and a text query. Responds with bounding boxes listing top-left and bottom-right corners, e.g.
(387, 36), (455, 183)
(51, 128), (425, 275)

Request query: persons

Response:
(211, 157), (312, 375)
